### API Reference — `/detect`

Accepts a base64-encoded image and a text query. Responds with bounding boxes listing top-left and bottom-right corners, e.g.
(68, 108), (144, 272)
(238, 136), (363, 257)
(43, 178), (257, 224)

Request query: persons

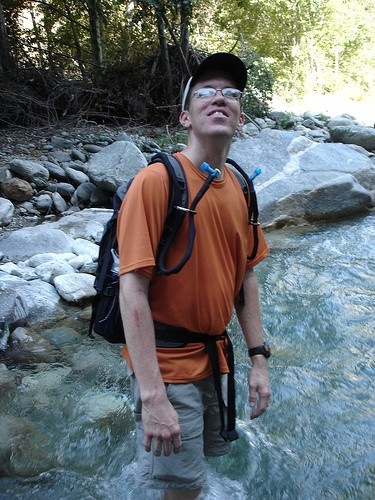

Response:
(114, 53), (274, 499)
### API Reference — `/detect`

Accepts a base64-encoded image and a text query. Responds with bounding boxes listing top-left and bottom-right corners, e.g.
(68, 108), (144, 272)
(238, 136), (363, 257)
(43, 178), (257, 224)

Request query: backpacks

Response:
(88, 153), (259, 344)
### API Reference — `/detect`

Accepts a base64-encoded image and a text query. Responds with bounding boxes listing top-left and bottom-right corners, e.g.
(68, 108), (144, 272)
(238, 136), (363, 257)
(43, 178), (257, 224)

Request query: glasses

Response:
(185, 87), (244, 114)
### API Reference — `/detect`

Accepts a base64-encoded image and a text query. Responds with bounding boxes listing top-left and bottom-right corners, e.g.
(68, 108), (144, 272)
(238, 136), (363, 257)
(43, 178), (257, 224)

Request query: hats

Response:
(181, 52), (247, 113)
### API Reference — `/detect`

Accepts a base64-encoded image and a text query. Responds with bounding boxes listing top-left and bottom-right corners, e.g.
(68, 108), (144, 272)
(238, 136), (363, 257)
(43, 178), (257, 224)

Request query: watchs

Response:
(248, 344), (272, 359)
